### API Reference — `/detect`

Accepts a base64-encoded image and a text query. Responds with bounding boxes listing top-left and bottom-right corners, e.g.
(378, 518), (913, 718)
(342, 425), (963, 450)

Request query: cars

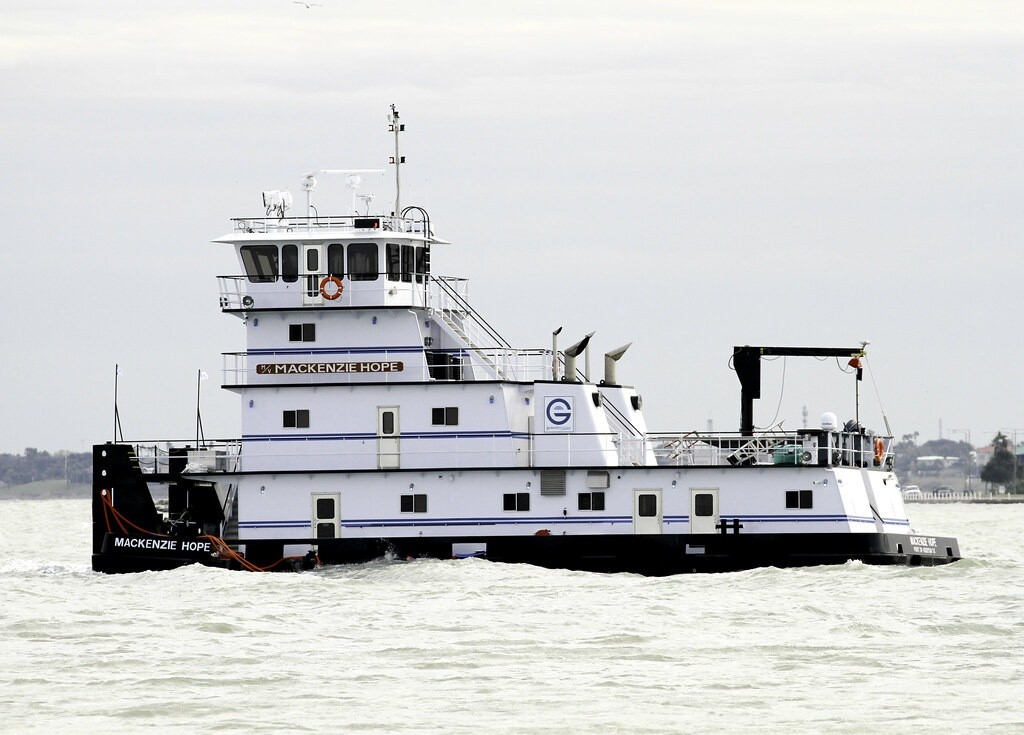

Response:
(933, 487), (954, 497)
(902, 485), (922, 499)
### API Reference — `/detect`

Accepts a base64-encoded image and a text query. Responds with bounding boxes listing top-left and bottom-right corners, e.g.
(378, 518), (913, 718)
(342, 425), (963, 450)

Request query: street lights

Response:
(953, 429), (972, 493)
(984, 427), (1024, 493)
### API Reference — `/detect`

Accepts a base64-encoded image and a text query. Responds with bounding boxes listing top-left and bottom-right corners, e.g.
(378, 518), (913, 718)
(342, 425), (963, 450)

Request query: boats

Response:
(93, 100), (965, 574)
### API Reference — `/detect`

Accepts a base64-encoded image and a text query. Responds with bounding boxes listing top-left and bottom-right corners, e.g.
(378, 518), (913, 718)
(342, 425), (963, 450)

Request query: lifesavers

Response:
(320, 277), (345, 300)
(874, 438), (885, 461)
